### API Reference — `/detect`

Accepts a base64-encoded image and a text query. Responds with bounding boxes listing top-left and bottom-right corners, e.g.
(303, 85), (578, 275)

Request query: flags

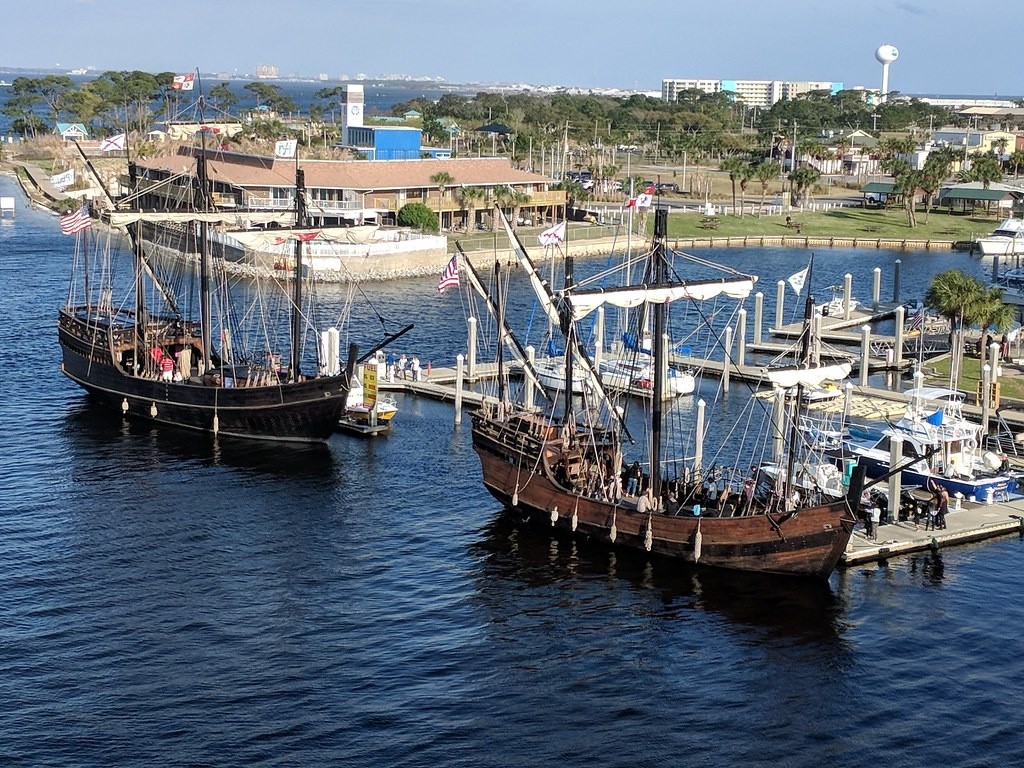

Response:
(60, 203), (92, 236)
(537, 221), (565, 246)
(275, 139), (297, 157)
(438, 255), (459, 294)
(787, 269), (808, 295)
(911, 306), (923, 328)
(101, 133), (125, 151)
(52, 169), (75, 187)
(626, 186), (655, 208)
(172, 73), (195, 90)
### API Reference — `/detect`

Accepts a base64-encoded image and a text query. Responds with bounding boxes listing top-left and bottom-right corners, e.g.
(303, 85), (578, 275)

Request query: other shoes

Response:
(943, 527), (946, 529)
(631, 494), (634, 498)
(939, 528), (943, 530)
(625, 493), (628, 497)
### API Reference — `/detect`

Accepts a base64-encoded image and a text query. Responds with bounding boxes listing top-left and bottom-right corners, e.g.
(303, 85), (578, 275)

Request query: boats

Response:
(758, 413), (890, 507)
(750, 390), (907, 422)
(976, 219), (1024, 256)
(815, 285), (861, 320)
(344, 375), (399, 426)
(796, 305), (1016, 502)
(775, 388), (843, 404)
(986, 268), (1024, 305)
(534, 362), (594, 395)
(573, 355), (695, 401)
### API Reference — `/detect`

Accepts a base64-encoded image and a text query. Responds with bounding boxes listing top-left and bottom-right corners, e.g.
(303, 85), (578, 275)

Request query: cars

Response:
(572, 178), (679, 190)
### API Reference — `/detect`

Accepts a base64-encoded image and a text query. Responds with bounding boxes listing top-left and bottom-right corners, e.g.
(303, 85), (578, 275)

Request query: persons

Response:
(410, 356), (420, 381)
(913, 480), (950, 531)
(625, 461), (640, 497)
(751, 466), (766, 494)
(636, 491), (651, 513)
(152, 345), (173, 383)
(976, 335), (993, 352)
(1002, 333), (1007, 345)
(796, 224), (801, 234)
(388, 351), (397, 366)
(706, 477), (717, 509)
(786, 215), (791, 224)
(663, 492), (679, 515)
(399, 354), (408, 379)
(864, 504), (881, 542)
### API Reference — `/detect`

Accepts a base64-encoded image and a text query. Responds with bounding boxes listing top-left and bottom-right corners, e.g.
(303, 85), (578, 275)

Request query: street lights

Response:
(682, 151), (687, 190)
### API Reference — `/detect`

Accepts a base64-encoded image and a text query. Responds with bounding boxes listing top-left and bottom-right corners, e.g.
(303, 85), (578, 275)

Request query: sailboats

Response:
(56, 66), (414, 452)
(455, 202), (867, 584)
(508, 177), (692, 378)
